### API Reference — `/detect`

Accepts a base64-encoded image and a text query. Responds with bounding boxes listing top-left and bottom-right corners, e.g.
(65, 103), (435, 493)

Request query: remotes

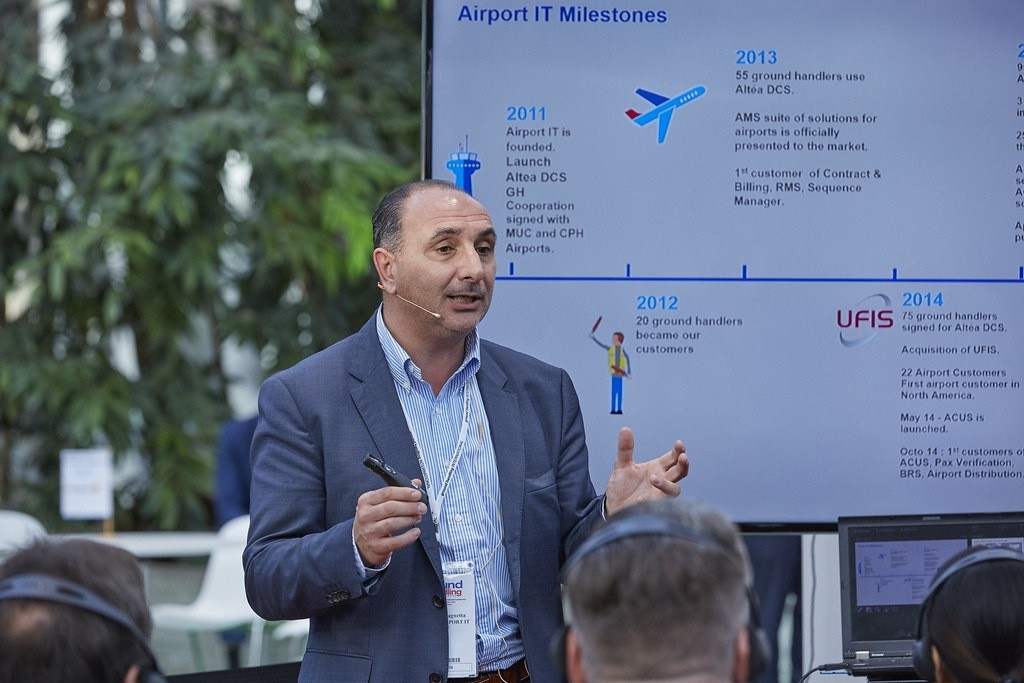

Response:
(363, 453), (427, 503)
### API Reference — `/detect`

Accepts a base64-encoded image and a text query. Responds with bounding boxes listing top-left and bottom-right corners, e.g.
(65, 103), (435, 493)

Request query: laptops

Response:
(837, 511), (1024, 676)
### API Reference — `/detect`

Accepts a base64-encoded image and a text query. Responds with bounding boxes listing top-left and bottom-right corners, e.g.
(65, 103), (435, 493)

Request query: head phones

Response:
(0, 575), (164, 683)
(550, 517), (771, 683)
(912, 549), (1024, 683)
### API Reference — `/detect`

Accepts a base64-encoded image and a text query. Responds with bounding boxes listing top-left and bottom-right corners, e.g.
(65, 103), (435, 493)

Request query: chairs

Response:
(151, 514), (258, 671)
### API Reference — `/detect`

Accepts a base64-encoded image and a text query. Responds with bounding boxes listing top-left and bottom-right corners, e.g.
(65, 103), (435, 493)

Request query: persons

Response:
(0, 537), (153, 683)
(565, 499), (754, 683)
(918, 548), (1024, 683)
(215, 411), (258, 670)
(242, 180), (688, 683)
(742, 533), (802, 682)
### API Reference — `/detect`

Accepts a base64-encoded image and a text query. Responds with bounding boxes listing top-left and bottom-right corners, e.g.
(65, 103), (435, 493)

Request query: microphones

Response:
(396, 294), (441, 319)
